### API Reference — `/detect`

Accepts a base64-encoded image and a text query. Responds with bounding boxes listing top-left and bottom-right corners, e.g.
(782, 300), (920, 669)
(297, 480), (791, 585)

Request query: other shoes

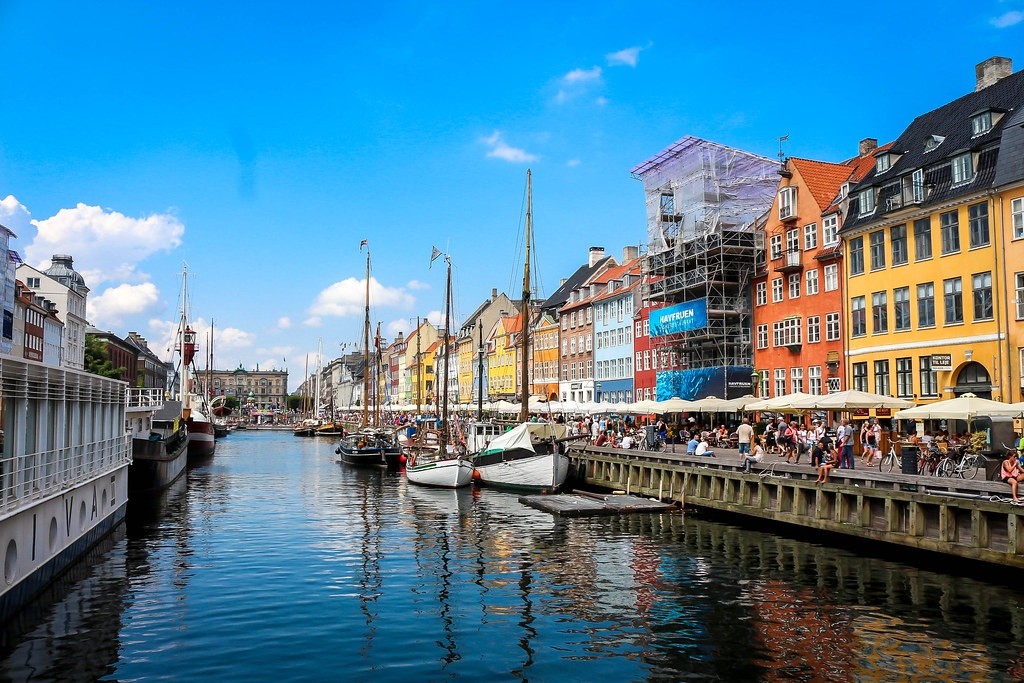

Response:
(742, 463), (745, 466)
(766, 451), (777, 454)
(783, 451), (787, 458)
(742, 470), (750, 474)
(867, 462), (875, 467)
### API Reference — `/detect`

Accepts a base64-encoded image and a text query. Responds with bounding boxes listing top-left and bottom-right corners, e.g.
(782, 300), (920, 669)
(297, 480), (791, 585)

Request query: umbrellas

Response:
(894, 393), (1024, 449)
(791, 389), (916, 430)
(337, 385), (824, 429)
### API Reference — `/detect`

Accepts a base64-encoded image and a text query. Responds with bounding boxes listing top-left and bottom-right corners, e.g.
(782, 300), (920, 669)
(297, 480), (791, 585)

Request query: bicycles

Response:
(878, 436), (1017, 482)
(636, 430), (666, 454)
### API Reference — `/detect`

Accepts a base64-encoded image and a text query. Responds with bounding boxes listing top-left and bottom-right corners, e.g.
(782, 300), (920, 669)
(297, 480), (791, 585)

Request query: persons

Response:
(321, 410), (1024, 503)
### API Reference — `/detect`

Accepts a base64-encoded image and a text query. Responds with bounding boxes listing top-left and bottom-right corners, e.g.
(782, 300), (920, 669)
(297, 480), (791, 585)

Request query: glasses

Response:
(871, 425), (875, 430)
(1010, 453), (1019, 462)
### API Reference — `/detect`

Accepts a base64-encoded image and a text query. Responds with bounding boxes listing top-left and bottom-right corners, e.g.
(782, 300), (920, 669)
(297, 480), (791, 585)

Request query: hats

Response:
(778, 416), (784, 422)
(790, 421), (797, 425)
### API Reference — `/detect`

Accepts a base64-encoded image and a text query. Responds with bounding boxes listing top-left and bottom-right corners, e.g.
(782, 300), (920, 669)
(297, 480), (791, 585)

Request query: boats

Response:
(126, 400), (192, 461)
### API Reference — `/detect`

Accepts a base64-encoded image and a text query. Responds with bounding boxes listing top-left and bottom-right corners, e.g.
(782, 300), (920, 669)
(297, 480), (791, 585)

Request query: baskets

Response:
(948, 450), (962, 461)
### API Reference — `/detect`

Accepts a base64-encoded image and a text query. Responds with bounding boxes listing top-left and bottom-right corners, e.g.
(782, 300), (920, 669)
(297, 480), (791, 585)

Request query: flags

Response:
(429, 245), (442, 269)
(359, 239), (368, 254)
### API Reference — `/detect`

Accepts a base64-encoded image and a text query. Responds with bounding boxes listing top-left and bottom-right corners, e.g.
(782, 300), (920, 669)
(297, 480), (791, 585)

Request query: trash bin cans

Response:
(902, 445), (919, 475)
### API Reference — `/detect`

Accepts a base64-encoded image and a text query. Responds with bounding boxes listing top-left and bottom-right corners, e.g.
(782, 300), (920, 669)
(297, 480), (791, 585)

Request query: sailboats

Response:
(203, 318), (228, 437)
(164, 260), (216, 458)
(293, 167), (592, 490)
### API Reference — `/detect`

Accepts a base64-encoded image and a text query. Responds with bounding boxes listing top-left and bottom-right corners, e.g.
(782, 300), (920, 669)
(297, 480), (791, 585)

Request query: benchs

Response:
(895, 441), (918, 459)
(919, 442), (956, 462)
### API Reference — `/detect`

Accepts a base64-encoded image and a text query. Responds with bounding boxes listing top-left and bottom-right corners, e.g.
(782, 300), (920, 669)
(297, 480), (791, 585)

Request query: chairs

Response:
(701, 431), (709, 441)
(708, 433), (717, 447)
(760, 435), (770, 455)
(730, 433), (739, 449)
